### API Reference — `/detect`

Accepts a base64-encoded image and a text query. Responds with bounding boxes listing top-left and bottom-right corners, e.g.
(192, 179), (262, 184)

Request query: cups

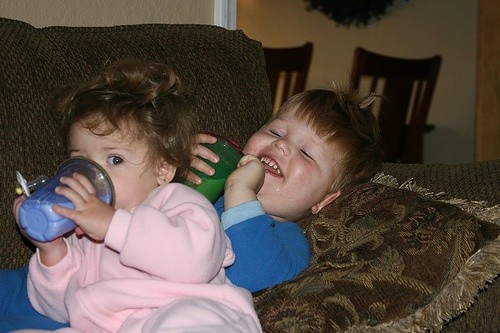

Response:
(19, 156), (116, 242)
(183, 131), (245, 204)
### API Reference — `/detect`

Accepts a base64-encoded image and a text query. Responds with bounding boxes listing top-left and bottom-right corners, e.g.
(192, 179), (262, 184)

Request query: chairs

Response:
(349, 46), (442, 163)
(263, 41), (314, 113)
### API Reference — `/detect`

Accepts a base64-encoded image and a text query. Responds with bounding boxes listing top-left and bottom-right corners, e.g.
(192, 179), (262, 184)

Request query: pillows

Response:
(251, 172), (500, 332)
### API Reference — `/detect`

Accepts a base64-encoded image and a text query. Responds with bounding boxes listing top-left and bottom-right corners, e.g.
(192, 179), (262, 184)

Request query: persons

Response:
(1, 82), (382, 331)
(9, 55), (269, 333)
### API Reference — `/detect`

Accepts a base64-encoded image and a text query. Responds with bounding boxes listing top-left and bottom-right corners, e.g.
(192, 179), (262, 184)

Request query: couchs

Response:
(0, 17), (500, 332)
